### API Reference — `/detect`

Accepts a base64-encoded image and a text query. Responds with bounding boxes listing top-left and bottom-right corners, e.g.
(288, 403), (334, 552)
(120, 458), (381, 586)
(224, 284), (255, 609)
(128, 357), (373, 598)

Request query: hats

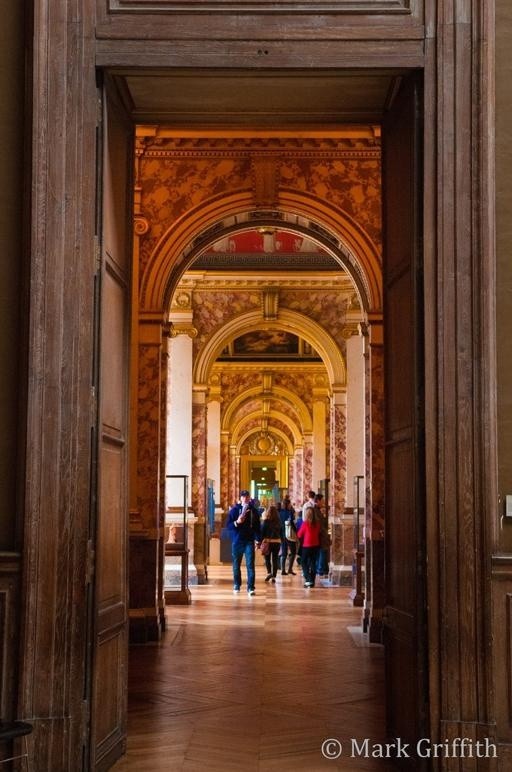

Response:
(240, 490), (249, 496)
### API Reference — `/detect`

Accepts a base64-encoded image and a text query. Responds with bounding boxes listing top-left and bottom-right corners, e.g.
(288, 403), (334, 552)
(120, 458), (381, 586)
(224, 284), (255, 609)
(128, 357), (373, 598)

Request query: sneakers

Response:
(281, 571), (288, 575)
(233, 584), (240, 593)
(304, 581), (313, 586)
(265, 573), (272, 581)
(272, 577), (276, 583)
(247, 589), (255, 595)
(288, 570), (296, 575)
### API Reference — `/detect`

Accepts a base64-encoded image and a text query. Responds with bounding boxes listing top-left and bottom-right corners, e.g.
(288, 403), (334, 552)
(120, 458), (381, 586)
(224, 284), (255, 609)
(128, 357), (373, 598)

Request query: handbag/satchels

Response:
(284, 512), (298, 543)
(260, 542), (270, 556)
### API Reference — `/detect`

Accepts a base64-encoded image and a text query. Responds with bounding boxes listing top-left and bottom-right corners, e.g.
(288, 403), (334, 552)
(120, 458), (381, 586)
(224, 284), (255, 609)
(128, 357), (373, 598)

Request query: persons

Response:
(232, 596), (255, 664)
(254, 491), (328, 588)
(227, 490), (262, 595)
